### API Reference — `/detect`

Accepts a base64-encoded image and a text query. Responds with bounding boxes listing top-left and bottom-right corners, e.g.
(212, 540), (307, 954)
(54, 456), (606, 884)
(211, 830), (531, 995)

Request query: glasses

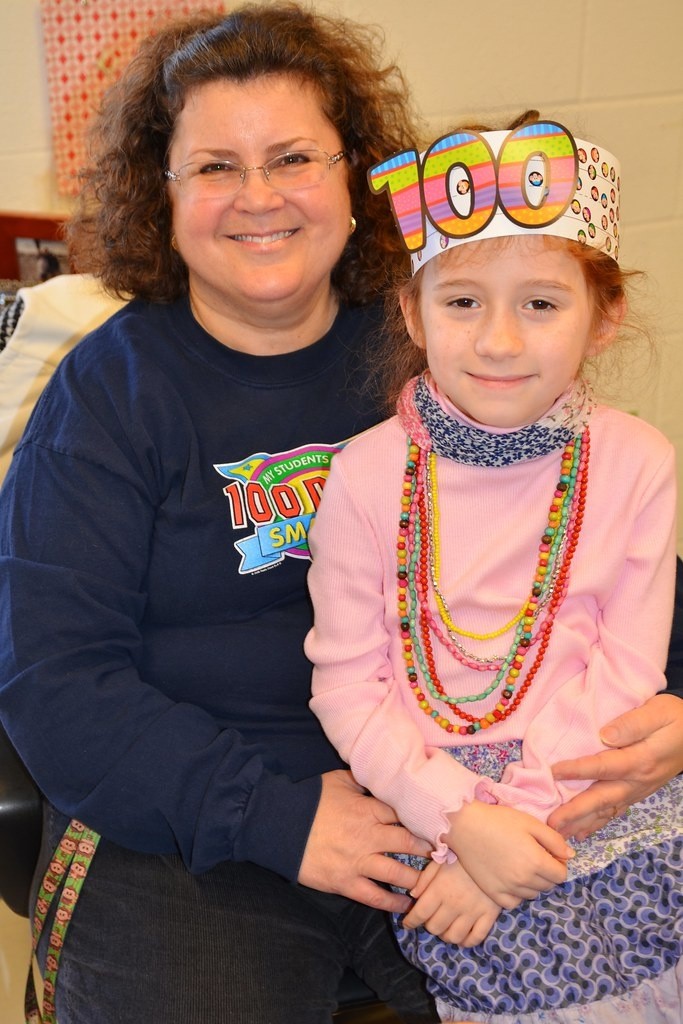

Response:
(160, 147), (347, 202)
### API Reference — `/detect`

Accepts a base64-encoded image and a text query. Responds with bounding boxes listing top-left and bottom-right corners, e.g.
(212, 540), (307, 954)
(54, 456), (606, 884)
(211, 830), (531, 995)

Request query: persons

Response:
(1, 0), (683, 1024)
(295, 109), (683, 1024)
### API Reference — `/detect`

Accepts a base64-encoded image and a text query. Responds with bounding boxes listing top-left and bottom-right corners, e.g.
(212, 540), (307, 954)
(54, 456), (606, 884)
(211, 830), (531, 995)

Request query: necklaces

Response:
(395, 420), (589, 737)
